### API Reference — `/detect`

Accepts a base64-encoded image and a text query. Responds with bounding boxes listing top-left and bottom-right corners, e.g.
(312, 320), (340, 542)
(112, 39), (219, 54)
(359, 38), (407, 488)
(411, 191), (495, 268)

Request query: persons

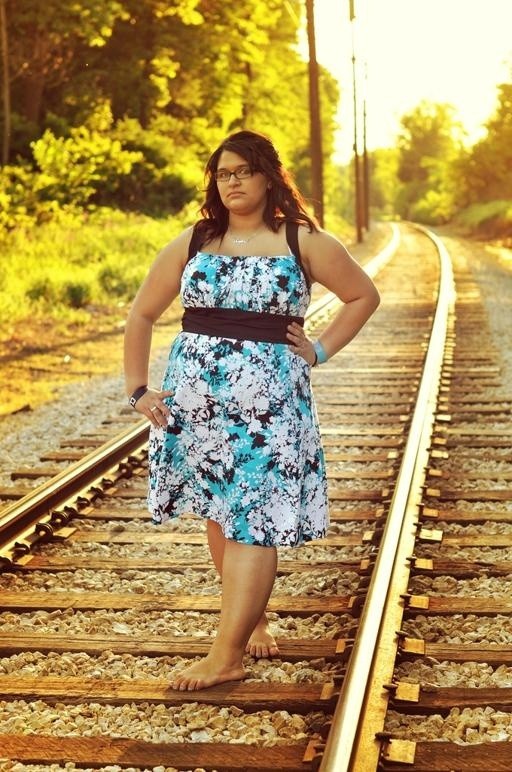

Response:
(124, 129), (381, 696)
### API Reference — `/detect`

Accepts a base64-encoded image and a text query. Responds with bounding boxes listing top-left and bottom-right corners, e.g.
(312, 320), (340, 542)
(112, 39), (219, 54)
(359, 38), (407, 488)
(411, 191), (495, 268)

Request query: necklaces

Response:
(224, 222), (267, 245)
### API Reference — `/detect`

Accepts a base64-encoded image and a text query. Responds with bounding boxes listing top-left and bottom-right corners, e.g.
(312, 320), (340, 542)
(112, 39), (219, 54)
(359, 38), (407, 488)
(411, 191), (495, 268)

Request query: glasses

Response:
(214, 166), (262, 181)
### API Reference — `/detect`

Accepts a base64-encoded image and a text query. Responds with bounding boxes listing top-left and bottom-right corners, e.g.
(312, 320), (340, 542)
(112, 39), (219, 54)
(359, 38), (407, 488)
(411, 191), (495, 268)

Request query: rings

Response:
(150, 405), (158, 412)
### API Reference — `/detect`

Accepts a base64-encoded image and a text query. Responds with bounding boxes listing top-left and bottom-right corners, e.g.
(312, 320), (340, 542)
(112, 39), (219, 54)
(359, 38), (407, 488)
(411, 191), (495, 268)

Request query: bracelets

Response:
(129, 384), (148, 409)
(312, 352), (318, 367)
(312, 340), (328, 365)
